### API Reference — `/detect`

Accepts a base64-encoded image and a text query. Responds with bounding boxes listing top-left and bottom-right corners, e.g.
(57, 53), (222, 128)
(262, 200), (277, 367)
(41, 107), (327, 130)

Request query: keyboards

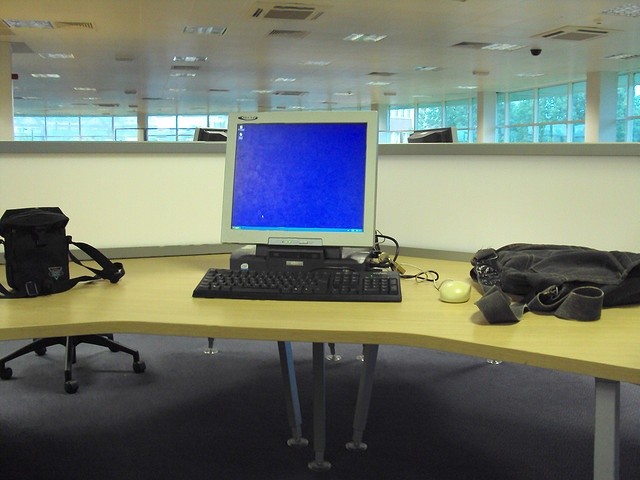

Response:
(191, 267), (403, 303)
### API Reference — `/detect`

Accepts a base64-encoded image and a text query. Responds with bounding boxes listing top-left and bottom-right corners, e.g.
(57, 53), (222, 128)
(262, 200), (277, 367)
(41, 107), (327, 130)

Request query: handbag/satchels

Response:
(0, 207), (125, 298)
(470, 243), (640, 324)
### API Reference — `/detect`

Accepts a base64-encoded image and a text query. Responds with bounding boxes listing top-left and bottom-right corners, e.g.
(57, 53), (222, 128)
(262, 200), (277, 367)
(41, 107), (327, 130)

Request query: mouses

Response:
(438, 279), (472, 304)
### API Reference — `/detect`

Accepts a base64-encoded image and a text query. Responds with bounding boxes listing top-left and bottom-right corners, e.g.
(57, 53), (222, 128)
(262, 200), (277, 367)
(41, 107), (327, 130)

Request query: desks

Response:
(0, 141), (639, 480)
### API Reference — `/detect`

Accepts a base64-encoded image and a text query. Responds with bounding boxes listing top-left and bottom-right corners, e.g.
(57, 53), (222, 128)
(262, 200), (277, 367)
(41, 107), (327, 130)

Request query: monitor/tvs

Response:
(220, 109), (379, 250)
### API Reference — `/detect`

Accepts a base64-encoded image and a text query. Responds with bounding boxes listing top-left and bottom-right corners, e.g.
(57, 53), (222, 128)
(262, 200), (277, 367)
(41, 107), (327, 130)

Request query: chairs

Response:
(0, 332), (147, 395)
